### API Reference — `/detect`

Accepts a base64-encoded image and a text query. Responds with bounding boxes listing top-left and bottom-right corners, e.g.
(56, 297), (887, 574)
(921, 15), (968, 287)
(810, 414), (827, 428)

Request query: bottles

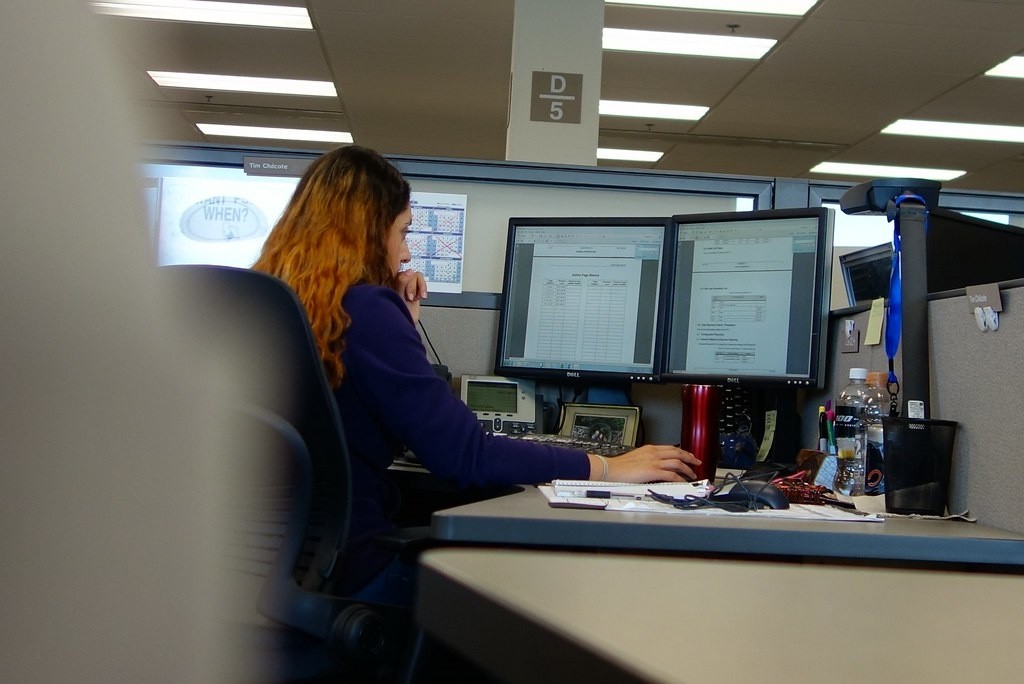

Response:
(832, 367), (869, 496)
(856, 372), (892, 497)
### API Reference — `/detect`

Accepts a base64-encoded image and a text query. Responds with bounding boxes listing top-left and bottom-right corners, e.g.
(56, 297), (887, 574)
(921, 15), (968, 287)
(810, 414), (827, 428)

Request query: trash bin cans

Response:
(882, 416), (958, 519)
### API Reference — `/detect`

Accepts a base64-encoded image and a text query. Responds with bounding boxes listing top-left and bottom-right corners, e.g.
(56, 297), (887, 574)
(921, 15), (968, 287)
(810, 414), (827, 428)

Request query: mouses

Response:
(710, 479), (791, 511)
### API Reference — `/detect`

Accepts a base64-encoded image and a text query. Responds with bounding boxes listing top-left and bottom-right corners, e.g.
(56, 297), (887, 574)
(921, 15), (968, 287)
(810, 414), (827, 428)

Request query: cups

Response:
(795, 448), (827, 481)
(880, 415), (959, 517)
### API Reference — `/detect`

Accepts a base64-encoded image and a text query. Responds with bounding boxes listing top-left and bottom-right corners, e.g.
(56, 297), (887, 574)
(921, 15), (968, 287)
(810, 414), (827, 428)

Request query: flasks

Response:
(680, 383), (724, 485)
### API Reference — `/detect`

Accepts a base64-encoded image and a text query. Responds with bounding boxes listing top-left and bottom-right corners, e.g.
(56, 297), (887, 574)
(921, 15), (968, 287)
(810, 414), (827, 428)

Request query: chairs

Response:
(165, 264), (474, 684)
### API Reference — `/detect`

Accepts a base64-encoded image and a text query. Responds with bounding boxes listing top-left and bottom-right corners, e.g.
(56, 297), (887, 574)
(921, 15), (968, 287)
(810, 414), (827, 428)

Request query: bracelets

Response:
(595, 454), (608, 482)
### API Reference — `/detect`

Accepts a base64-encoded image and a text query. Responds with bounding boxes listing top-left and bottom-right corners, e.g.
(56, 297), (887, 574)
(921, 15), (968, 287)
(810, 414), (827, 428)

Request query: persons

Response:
(247, 145), (701, 684)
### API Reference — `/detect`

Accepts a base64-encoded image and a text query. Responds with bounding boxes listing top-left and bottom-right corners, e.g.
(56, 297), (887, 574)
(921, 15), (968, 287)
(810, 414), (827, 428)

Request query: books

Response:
(540, 479), (696, 500)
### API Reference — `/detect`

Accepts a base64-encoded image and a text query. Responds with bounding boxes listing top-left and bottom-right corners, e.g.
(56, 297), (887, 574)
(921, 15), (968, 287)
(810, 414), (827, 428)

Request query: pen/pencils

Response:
(557, 490), (651, 500)
(816, 400), (835, 454)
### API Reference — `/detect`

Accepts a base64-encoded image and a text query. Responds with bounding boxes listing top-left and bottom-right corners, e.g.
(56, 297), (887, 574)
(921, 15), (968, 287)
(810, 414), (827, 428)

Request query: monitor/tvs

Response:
(494, 217), (674, 407)
(659, 208), (836, 468)
(838, 242), (895, 306)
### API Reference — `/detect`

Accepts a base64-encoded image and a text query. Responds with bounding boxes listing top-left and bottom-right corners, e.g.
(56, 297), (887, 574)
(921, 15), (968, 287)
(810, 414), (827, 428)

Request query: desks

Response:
(384, 447), (1024, 684)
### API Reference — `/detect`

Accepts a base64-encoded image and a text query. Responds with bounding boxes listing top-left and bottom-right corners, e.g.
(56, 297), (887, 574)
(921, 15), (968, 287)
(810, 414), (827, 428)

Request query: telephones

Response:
(459, 372), (544, 436)
